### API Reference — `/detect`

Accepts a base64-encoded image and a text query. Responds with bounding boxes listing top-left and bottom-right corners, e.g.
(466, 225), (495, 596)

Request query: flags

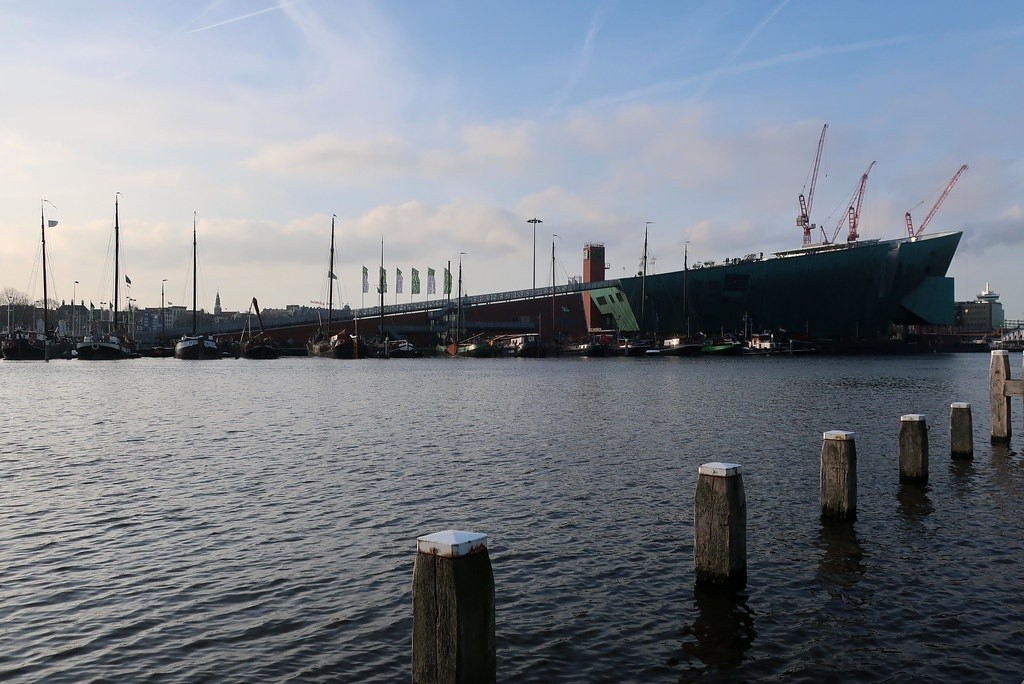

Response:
(396, 268), (404, 294)
(362, 266), (369, 294)
(411, 267), (420, 295)
(427, 267), (436, 294)
(378, 266), (388, 293)
(442, 268), (453, 294)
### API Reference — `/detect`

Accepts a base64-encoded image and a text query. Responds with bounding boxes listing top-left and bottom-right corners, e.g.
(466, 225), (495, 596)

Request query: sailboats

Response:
(1, 197), (73, 363)
(433, 251), (501, 358)
(537, 222), (702, 358)
(172, 209), (226, 359)
(305, 212), (354, 358)
(77, 192), (144, 361)
(233, 297), (279, 359)
(361, 234), (399, 359)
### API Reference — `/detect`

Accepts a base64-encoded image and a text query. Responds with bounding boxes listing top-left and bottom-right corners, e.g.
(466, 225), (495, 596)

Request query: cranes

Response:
(820, 161), (874, 249)
(795, 123), (828, 251)
(903, 162), (969, 244)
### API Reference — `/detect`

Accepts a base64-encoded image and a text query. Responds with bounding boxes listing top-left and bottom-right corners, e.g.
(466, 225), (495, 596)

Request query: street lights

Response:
(526, 218), (543, 296)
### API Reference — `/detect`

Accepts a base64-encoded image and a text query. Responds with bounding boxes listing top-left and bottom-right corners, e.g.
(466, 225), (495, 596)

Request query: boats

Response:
(391, 340), (426, 358)
(698, 311), (825, 358)
(503, 315), (554, 358)
